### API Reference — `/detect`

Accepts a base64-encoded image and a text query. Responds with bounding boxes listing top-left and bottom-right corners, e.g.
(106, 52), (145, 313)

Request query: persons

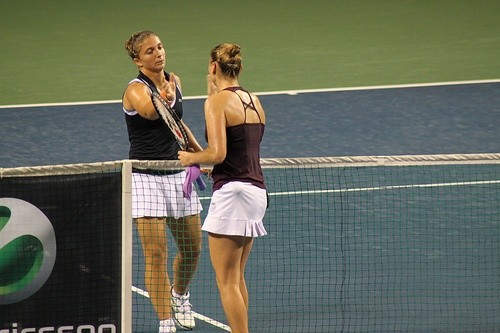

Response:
(168, 42), (270, 333)
(122, 30), (205, 333)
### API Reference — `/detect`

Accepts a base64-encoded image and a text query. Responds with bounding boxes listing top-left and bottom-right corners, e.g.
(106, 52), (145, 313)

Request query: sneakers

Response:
(158, 317), (177, 333)
(168, 284), (196, 331)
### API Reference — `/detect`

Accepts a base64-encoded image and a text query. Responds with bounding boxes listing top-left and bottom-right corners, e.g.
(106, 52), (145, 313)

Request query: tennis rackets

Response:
(149, 93), (208, 192)
